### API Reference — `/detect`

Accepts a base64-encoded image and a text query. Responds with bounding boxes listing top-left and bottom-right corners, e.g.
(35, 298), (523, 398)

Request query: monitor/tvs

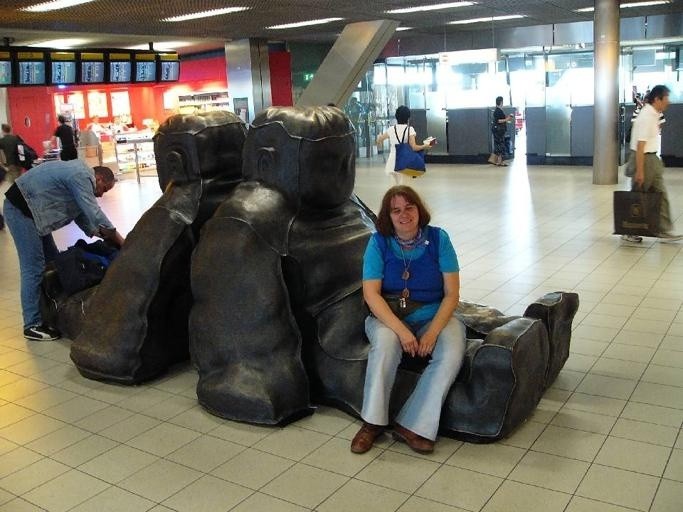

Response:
(16, 59), (50, 87)
(50, 59), (79, 86)
(0, 59), (15, 87)
(79, 60), (108, 84)
(134, 60), (159, 83)
(159, 60), (181, 83)
(108, 60), (134, 84)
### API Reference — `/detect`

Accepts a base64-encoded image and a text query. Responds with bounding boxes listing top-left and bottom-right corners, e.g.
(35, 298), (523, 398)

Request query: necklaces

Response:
(398, 240), (418, 309)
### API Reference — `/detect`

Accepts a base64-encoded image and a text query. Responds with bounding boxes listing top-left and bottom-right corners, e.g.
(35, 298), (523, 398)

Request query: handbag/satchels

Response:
(45, 239), (117, 297)
(612, 182), (663, 238)
(365, 294), (423, 321)
(395, 127), (426, 179)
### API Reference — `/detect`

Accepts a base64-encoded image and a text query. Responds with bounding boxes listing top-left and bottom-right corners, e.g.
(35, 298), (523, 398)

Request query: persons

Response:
(1, 123), (36, 184)
(350, 186), (468, 458)
(55, 114), (78, 161)
(346, 96), (398, 147)
(110, 115), (129, 132)
(90, 113), (104, 132)
(619, 85), (683, 243)
(487, 96), (513, 166)
(376, 105), (432, 186)
(2, 157), (125, 340)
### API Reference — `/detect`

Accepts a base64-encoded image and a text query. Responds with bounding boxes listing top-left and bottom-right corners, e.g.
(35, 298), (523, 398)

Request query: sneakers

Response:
(23, 320), (61, 342)
(620, 234), (643, 243)
(659, 232), (683, 242)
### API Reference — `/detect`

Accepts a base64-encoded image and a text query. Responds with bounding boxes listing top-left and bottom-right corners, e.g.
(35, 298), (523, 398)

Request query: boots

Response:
(497, 156), (508, 166)
(488, 153), (498, 165)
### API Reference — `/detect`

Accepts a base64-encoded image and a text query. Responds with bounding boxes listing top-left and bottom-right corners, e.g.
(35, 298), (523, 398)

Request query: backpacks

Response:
(16, 134), (38, 169)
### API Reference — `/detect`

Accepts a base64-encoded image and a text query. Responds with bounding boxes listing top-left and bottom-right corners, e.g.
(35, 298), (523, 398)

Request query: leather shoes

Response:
(351, 421), (385, 454)
(392, 422), (435, 455)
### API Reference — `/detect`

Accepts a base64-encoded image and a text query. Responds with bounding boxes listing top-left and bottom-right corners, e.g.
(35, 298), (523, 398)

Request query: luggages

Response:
(39, 238), (121, 329)
